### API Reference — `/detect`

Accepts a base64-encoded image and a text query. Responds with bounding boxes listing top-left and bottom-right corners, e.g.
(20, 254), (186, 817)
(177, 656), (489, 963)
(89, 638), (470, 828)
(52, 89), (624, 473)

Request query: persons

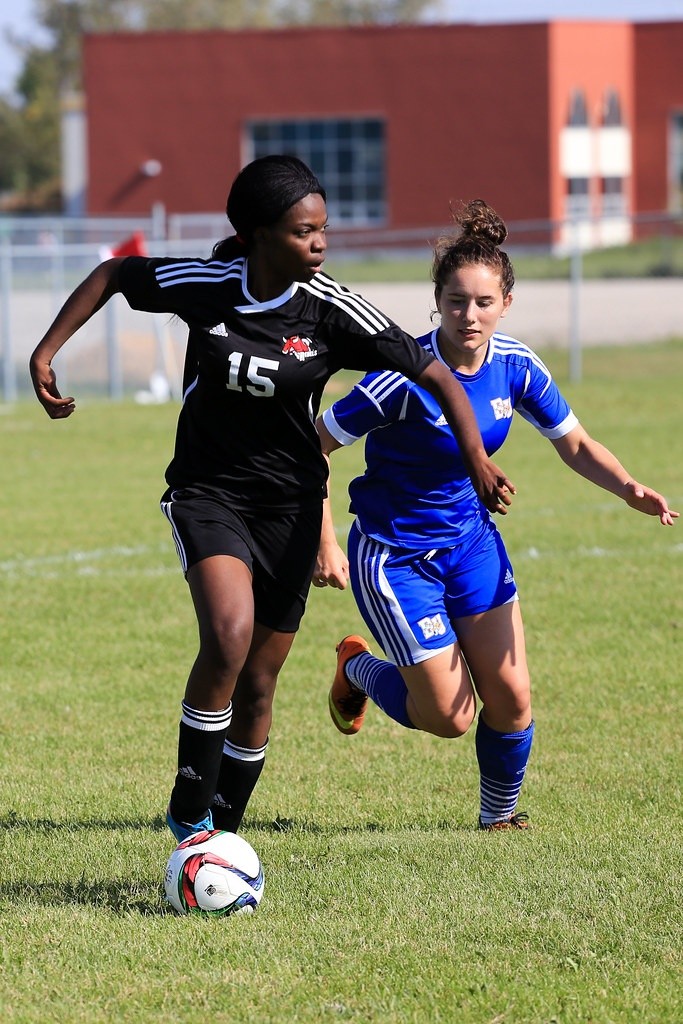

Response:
(313, 196), (681, 830)
(30, 154), (518, 841)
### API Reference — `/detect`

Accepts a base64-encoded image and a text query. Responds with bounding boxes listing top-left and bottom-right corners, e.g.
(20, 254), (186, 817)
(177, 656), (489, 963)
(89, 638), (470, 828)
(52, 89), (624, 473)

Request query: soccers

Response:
(164, 828), (266, 918)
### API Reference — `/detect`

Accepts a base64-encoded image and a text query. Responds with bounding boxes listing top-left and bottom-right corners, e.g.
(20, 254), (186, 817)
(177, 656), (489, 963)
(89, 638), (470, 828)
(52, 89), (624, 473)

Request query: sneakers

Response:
(479, 815), (530, 834)
(330, 635), (372, 734)
(165, 801), (212, 843)
